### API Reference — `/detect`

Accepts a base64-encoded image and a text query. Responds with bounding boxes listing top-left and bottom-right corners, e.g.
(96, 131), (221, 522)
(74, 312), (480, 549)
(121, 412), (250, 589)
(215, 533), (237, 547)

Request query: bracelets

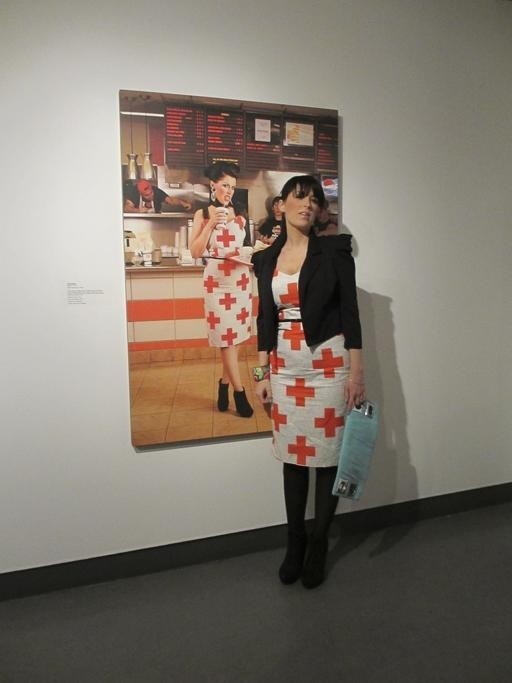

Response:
(257, 365), (270, 380)
(349, 376), (364, 383)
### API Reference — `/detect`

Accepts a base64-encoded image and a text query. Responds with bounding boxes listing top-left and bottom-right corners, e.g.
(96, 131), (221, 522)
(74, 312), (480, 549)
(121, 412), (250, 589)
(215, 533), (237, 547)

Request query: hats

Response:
(135, 180), (152, 194)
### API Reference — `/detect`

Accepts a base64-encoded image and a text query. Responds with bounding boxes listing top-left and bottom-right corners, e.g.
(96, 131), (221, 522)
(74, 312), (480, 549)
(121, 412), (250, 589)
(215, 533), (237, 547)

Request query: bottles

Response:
(125, 151), (155, 186)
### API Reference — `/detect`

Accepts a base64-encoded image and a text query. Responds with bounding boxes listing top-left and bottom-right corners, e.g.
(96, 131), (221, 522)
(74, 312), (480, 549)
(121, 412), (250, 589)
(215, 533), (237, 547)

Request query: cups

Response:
(238, 245), (252, 258)
(130, 245), (162, 267)
(214, 205), (229, 230)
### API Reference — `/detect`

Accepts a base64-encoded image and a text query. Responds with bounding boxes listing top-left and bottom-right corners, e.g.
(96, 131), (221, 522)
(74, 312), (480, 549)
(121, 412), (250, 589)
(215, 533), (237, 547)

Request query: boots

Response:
(299, 528), (330, 590)
(215, 377), (230, 412)
(277, 526), (307, 584)
(232, 386), (255, 417)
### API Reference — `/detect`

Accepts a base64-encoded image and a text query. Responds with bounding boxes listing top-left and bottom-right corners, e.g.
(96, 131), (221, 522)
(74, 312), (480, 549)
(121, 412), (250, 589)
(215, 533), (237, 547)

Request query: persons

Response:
(186, 159), (259, 417)
(255, 195), (283, 245)
(313, 199), (337, 238)
(254, 174), (367, 589)
(123, 178), (193, 216)
